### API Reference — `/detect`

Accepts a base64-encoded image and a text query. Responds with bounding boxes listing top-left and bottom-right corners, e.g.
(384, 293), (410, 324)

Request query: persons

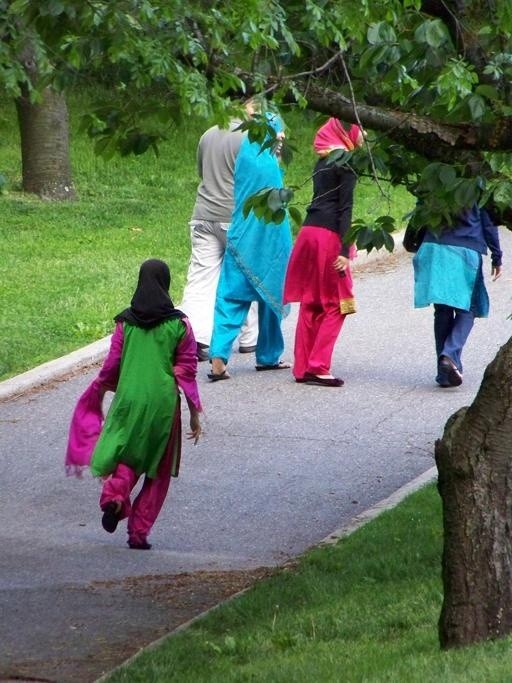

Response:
(283, 115), (369, 388)
(63, 258), (202, 549)
(206, 112), (294, 380)
(403, 177), (503, 388)
(179, 95), (260, 362)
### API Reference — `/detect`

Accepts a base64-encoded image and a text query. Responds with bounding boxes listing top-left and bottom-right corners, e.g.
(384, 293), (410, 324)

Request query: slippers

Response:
(255, 360), (291, 372)
(207, 370), (230, 379)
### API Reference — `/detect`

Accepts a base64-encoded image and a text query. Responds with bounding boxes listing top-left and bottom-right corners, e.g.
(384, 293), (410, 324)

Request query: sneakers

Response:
(130, 541), (153, 548)
(196, 346), (208, 360)
(296, 371), (344, 386)
(436, 357), (462, 387)
(240, 346), (258, 353)
(102, 500), (122, 533)
